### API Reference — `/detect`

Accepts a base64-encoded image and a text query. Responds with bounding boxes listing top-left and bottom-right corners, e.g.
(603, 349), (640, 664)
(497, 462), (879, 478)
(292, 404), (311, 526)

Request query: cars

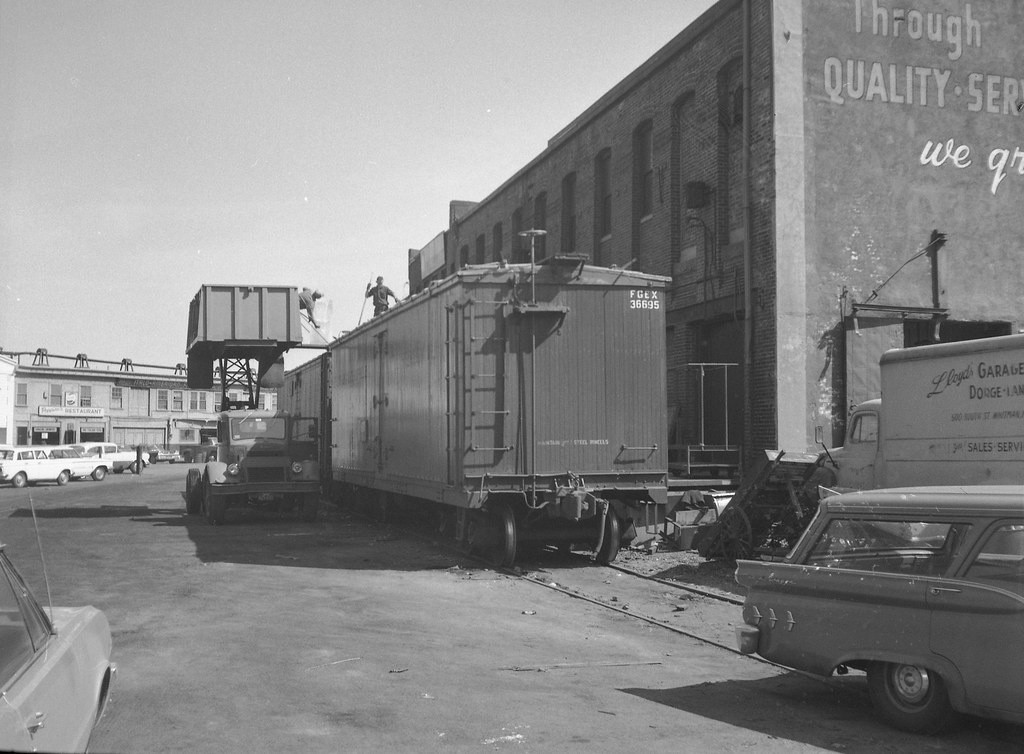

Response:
(0, 441), (180, 488)
(1, 540), (120, 753)
(735, 485), (1024, 731)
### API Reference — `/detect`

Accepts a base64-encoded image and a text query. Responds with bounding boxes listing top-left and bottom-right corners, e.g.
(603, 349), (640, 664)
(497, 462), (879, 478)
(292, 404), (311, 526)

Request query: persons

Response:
(299, 287), (324, 328)
(365, 276), (401, 317)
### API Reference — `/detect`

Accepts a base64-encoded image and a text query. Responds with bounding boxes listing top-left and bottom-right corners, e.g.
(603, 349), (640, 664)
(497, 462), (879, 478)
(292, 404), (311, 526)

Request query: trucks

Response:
(817, 330), (1024, 496)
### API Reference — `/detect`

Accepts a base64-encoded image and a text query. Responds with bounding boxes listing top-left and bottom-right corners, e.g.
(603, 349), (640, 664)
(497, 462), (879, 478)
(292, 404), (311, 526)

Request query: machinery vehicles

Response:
(185, 283), (323, 523)
